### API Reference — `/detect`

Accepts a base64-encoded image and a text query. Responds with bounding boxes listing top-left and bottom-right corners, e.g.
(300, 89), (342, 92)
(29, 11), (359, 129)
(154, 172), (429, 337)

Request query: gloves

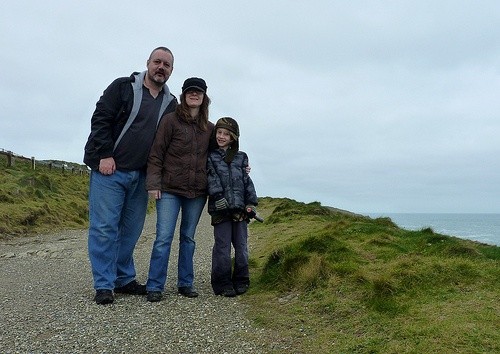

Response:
(213, 194), (230, 211)
(245, 204), (256, 217)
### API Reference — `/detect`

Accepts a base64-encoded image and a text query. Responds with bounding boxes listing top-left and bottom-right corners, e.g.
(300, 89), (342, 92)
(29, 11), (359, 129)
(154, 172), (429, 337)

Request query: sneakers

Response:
(147, 291), (163, 302)
(114, 280), (149, 295)
(178, 287), (198, 297)
(95, 289), (114, 304)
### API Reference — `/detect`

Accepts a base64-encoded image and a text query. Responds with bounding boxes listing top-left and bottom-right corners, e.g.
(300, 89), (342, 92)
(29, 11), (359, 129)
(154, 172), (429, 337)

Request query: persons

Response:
(207, 117), (258, 298)
(83, 47), (179, 305)
(145, 77), (250, 302)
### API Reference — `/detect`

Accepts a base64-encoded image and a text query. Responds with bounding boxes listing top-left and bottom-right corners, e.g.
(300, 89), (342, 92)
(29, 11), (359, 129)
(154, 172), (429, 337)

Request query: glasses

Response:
(185, 88), (203, 95)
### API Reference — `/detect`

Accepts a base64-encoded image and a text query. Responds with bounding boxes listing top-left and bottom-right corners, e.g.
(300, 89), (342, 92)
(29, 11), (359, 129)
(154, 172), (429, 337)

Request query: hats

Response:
(182, 77), (207, 93)
(215, 117), (240, 136)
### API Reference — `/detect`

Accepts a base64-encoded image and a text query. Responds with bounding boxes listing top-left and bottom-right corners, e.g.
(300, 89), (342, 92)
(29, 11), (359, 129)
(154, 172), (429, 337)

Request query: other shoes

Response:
(219, 283), (249, 297)
